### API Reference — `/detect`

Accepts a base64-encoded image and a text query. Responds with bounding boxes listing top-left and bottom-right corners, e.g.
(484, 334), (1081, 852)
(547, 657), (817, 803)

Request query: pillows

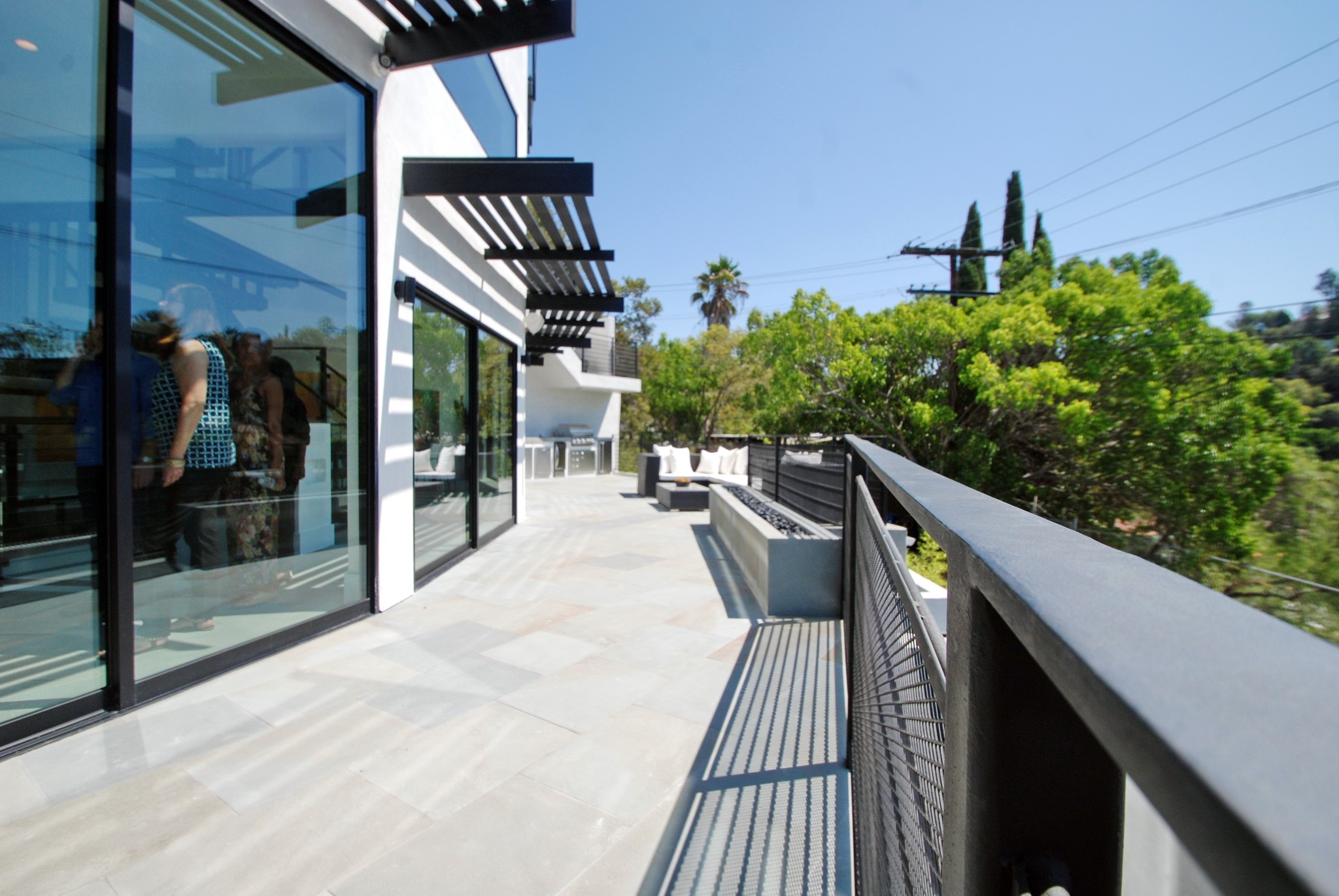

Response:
(653, 444), (673, 475)
(414, 448), (435, 473)
(733, 446), (748, 475)
(669, 446), (694, 474)
(717, 445), (739, 475)
(434, 444), (456, 472)
(694, 449), (722, 475)
(454, 445), (466, 456)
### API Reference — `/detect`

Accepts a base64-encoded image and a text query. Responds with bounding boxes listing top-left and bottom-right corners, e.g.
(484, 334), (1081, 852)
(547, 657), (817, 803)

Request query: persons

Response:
(53, 308), (162, 647)
(90, 282), (238, 661)
(230, 326), (284, 613)
(271, 355), (312, 585)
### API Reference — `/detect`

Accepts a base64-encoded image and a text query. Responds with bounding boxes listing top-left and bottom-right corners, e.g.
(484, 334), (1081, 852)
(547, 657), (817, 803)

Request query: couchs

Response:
(637, 444), (748, 497)
(413, 444), (469, 492)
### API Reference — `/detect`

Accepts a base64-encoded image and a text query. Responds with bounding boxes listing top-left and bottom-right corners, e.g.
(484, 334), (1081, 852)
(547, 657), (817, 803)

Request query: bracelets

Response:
(140, 456), (154, 463)
(165, 458), (185, 469)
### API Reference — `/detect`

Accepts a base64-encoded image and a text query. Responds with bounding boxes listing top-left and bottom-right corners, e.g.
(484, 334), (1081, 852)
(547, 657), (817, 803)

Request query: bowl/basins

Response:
(676, 481), (691, 487)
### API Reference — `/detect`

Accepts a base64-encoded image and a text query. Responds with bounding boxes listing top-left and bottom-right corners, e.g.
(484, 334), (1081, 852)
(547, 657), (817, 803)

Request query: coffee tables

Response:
(414, 481), (444, 508)
(655, 482), (710, 509)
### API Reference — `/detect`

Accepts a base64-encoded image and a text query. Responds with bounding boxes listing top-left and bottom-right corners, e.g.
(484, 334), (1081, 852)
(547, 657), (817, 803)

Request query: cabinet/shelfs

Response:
(525, 436), (612, 481)
